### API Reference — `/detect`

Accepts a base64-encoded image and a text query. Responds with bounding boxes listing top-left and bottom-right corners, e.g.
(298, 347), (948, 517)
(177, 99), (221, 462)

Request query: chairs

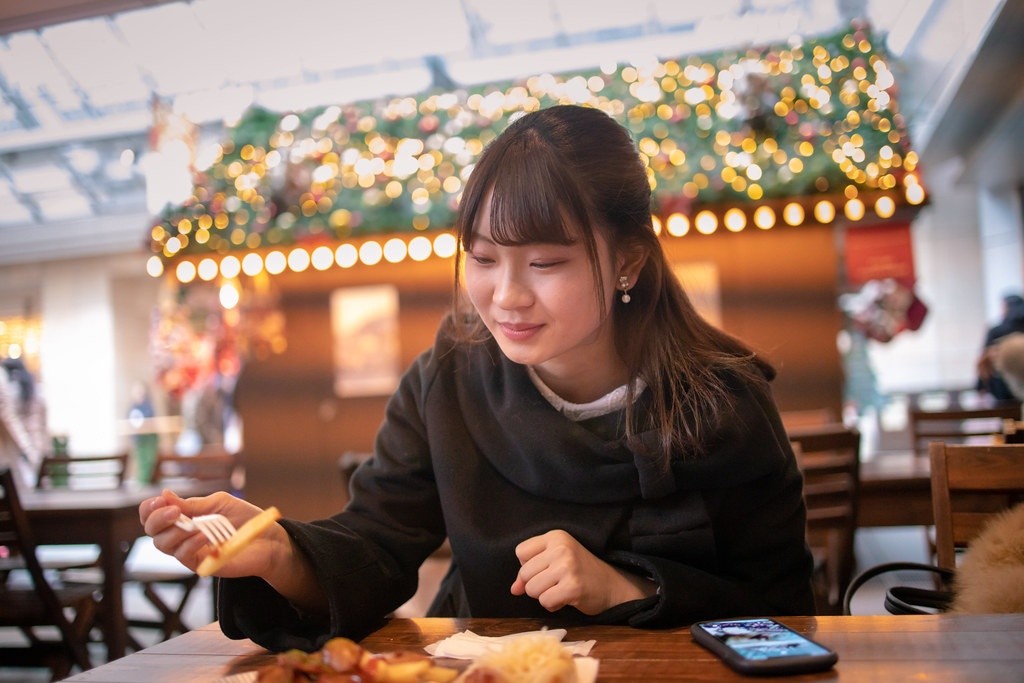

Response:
(0, 453), (240, 683)
(910, 405), (1024, 589)
(928, 441), (1024, 569)
(791, 424), (864, 612)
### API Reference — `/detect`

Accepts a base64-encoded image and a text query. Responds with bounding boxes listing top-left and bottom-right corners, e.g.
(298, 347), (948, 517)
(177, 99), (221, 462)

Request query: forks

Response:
(175, 513), (236, 545)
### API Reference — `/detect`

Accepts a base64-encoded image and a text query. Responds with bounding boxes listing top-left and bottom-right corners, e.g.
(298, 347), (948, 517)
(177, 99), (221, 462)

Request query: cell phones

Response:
(690, 617), (838, 677)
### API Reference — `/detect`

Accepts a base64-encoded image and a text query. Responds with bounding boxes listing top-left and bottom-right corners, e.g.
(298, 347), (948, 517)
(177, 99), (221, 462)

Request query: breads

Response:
(467, 636), (581, 683)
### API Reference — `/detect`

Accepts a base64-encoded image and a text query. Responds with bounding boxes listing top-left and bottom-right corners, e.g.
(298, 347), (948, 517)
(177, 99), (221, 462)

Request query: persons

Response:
(139, 106), (813, 652)
(974, 294), (1023, 443)
(0, 359), (49, 489)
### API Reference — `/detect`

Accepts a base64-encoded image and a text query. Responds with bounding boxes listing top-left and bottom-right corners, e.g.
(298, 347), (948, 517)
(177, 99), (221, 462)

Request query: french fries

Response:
(357, 650), (459, 683)
(197, 505), (282, 576)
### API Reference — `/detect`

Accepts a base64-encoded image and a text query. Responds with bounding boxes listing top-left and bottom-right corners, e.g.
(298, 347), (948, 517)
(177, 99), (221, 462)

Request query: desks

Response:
(0, 484), (227, 660)
(63, 614), (1024, 683)
(859, 451), (934, 527)
